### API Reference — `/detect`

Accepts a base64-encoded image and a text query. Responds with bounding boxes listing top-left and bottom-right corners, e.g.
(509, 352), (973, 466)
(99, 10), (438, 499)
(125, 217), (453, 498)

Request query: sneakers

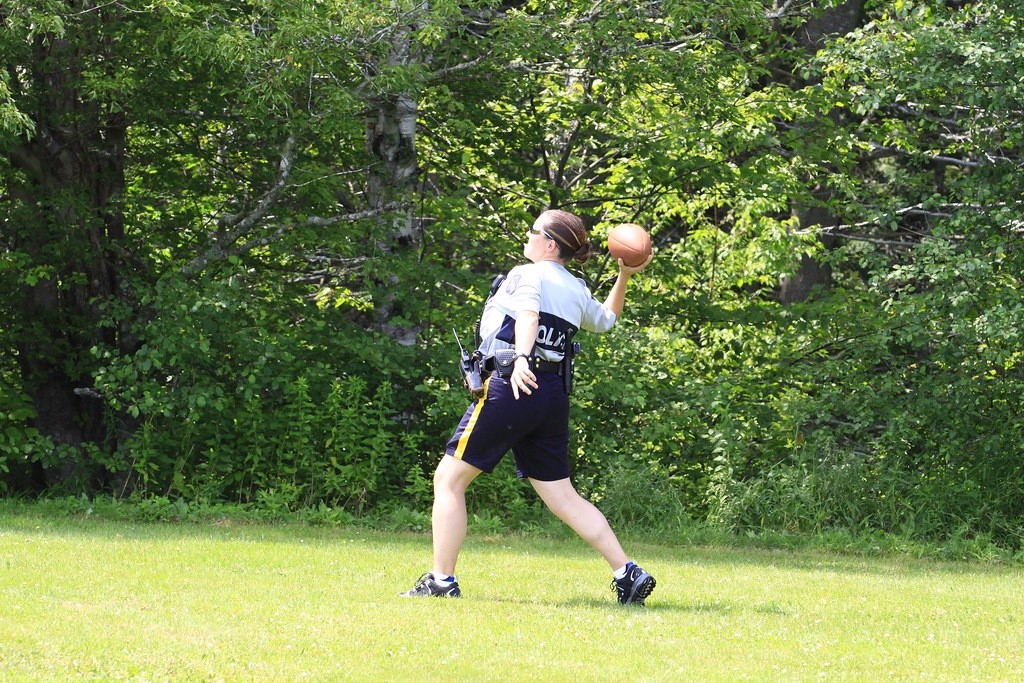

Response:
(611, 561), (656, 606)
(398, 572), (460, 596)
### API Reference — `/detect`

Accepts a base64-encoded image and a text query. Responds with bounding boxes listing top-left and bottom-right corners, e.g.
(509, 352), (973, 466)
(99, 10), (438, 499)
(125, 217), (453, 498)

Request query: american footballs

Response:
(608, 222), (652, 267)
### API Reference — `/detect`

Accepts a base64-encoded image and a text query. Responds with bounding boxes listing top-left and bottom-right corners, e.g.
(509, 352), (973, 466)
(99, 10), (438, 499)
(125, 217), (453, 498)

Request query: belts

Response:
(484, 357), (562, 375)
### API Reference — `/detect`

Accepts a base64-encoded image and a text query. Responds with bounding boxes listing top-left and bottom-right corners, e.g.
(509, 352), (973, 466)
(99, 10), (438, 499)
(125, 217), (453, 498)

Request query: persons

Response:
(400, 210), (658, 605)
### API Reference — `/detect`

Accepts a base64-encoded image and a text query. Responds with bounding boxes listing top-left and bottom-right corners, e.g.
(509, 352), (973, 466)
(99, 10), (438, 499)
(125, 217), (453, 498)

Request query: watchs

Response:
(512, 353), (529, 362)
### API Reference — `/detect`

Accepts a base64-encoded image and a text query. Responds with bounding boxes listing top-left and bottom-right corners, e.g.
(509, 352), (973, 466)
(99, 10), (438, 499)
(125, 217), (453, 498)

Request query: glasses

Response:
(530, 225), (560, 251)
